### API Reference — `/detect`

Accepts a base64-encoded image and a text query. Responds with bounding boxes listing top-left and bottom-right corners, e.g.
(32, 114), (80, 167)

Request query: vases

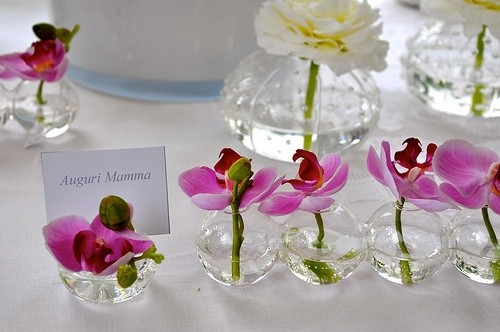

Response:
(398, 19), (500, 120)
(58, 233), (158, 305)
(445, 208), (500, 285)
(363, 201), (450, 286)
(194, 204), (280, 288)
(11, 78), (80, 140)
(220, 49), (382, 165)
(280, 200), (364, 286)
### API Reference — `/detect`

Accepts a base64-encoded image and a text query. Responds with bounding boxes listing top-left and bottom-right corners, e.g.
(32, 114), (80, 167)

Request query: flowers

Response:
(365, 136), (463, 214)
(176, 147), (287, 211)
(418, 0), (500, 42)
(254, 0), (391, 77)
(432, 139), (500, 216)
(0, 23), (80, 83)
(257, 148), (349, 216)
(42, 193), (166, 290)
